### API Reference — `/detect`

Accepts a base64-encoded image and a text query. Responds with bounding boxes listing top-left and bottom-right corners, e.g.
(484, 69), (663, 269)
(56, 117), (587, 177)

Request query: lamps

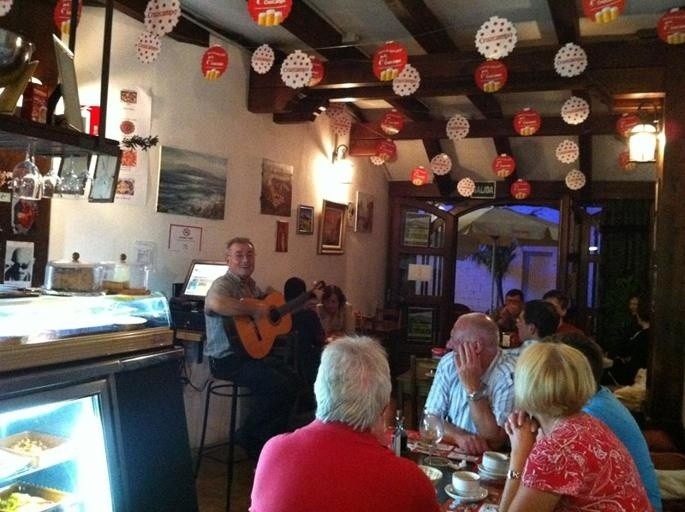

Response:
(627, 100), (663, 164)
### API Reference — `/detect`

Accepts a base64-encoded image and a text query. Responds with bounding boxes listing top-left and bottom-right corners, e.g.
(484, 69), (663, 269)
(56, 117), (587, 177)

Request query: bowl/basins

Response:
(417, 465), (442, 491)
(0, 27), (37, 88)
(482, 450), (508, 473)
(44, 263), (121, 295)
(451, 470), (481, 496)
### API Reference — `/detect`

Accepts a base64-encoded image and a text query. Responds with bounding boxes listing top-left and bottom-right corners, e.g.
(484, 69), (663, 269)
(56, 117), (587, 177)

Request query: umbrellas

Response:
(453, 205), (558, 314)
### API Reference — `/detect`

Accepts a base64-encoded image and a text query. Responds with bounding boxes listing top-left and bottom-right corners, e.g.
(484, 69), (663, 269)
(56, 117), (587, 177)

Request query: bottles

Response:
(392, 416), (408, 457)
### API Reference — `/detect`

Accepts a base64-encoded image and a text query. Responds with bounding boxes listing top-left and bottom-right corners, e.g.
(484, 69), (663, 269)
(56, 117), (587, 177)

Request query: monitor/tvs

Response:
(179, 259), (231, 298)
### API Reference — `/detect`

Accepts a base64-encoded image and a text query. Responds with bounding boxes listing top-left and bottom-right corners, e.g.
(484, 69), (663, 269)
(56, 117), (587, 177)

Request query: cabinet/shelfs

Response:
(0, 346), (200, 511)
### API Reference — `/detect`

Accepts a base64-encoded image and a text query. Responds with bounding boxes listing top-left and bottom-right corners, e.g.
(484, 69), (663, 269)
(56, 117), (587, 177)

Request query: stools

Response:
(193, 374), (267, 511)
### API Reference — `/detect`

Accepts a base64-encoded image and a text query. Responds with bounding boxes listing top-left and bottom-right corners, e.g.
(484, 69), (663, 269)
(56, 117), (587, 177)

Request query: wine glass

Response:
(10, 134), (116, 202)
(417, 408), (445, 468)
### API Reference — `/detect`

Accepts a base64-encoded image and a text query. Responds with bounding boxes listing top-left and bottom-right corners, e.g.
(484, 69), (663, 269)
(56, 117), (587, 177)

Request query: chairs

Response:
(409, 354), (440, 431)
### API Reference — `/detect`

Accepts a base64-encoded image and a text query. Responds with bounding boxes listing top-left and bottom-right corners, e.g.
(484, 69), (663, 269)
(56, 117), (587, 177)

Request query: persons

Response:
(304, 292), (319, 312)
(281, 276), (327, 348)
(201, 235), (279, 462)
(312, 282), (355, 336)
(416, 288), (665, 511)
(245, 332), (441, 512)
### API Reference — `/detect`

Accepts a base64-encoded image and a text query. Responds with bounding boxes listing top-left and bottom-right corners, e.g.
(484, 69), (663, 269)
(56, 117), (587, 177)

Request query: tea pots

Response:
(130, 240), (158, 292)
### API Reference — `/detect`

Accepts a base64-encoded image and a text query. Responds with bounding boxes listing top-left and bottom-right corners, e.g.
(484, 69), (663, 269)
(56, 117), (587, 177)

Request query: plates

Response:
(444, 485), (488, 503)
(477, 464), (509, 477)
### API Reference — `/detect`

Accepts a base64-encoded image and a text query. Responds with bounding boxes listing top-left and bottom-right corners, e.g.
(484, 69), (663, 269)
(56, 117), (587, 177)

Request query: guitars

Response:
(223, 280), (325, 360)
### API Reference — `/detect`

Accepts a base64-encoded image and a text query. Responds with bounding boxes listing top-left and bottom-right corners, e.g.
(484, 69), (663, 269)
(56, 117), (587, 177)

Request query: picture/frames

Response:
(296, 203), (315, 235)
(318, 199), (348, 256)
